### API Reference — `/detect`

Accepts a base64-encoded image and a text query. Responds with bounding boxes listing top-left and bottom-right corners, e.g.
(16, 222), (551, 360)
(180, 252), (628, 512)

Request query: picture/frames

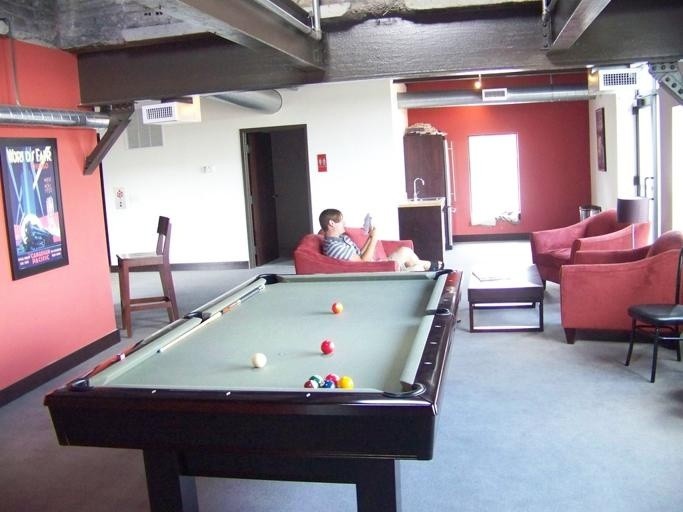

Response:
(0, 137), (69, 282)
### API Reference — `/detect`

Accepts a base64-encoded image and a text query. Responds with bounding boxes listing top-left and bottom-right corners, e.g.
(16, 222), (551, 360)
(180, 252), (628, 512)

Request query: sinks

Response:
(412, 198), (440, 201)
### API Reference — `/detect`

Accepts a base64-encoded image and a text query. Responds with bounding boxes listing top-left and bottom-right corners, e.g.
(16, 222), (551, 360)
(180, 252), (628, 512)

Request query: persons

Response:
(318, 208), (444, 271)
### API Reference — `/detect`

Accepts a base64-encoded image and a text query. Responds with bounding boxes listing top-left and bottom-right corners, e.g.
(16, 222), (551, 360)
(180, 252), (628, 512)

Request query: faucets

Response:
(413, 177), (424, 199)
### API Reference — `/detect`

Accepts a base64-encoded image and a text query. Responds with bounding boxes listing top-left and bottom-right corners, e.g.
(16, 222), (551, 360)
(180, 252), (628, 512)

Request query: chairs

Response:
(624, 249), (683, 382)
(530, 208), (683, 343)
(114, 216), (181, 340)
(292, 226), (414, 275)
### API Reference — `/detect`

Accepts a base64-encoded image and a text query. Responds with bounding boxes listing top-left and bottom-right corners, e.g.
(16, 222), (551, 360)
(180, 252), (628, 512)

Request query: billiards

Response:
(321, 341), (334, 354)
(253, 353), (266, 368)
(304, 374), (354, 387)
(332, 303), (344, 313)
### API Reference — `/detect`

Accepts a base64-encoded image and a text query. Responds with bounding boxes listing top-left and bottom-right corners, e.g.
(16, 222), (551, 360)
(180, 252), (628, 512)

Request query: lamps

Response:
(616, 197), (649, 250)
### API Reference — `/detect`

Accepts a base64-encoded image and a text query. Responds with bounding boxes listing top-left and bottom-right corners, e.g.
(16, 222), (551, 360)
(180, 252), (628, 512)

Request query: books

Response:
(472, 270), (499, 282)
(359, 212), (373, 236)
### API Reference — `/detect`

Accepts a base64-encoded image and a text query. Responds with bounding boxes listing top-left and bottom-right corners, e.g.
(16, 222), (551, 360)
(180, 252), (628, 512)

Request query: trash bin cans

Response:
(578, 205), (602, 222)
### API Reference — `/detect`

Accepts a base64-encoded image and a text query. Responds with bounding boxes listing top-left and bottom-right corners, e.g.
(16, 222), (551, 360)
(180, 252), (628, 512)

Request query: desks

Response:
(42, 268), (463, 512)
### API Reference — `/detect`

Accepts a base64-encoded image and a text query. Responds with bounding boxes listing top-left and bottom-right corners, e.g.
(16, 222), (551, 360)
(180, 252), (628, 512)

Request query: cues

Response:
(160, 285), (262, 353)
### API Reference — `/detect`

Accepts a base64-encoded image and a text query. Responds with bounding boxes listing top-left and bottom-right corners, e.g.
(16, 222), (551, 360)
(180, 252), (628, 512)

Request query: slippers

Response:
(421, 260), (444, 272)
